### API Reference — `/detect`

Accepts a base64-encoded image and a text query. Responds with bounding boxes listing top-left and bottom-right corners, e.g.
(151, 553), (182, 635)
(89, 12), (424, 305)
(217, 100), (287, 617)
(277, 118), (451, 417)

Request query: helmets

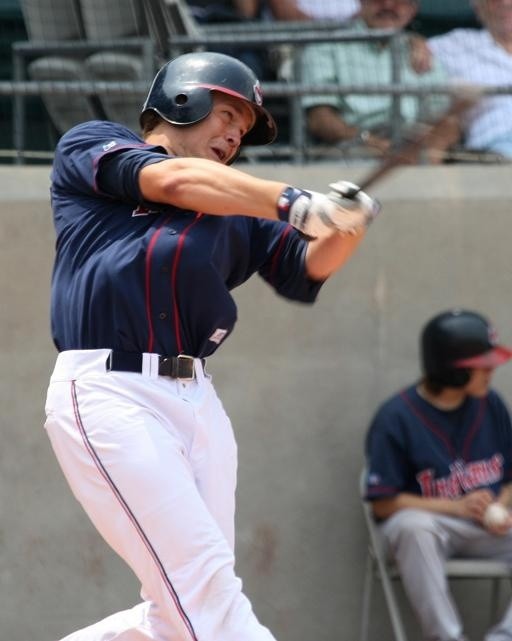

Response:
(421, 308), (511, 374)
(142, 50), (279, 146)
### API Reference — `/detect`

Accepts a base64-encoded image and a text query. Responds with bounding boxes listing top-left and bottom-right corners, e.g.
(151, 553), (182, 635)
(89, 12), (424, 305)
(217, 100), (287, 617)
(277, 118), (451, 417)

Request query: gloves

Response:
(278, 183), (335, 239)
(324, 182), (380, 235)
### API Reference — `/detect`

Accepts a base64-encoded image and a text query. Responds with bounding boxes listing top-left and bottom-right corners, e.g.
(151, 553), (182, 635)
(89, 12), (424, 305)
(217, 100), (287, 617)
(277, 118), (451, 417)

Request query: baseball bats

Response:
(341, 94), (488, 200)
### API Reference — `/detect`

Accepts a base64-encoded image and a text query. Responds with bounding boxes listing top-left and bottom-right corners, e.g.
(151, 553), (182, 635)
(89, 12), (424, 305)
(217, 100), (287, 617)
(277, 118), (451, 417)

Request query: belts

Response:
(105, 350), (209, 379)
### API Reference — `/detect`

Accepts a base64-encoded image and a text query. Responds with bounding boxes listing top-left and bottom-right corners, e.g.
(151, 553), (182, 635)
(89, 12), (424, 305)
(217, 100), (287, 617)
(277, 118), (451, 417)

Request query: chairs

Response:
(78, 1), (142, 41)
(22, 1), (82, 42)
(86, 49), (150, 139)
(357, 463), (511, 641)
(26, 55), (103, 153)
(161, 1), (479, 161)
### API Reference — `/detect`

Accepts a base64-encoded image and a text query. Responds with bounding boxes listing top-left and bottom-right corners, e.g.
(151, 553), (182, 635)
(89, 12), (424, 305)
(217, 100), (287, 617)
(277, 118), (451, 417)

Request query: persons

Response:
(354, 305), (511, 641)
(188, 0), (469, 162)
(409, 0), (512, 157)
(39, 50), (370, 641)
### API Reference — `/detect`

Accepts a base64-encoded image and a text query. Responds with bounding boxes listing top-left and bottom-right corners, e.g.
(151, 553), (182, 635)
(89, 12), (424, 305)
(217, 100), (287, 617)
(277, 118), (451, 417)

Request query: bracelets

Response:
(277, 184), (312, 223)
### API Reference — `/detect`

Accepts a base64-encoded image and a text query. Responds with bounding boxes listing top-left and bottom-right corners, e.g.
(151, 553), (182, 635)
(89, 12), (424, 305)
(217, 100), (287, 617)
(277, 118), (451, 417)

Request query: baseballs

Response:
(483, 503), (508, 527)
